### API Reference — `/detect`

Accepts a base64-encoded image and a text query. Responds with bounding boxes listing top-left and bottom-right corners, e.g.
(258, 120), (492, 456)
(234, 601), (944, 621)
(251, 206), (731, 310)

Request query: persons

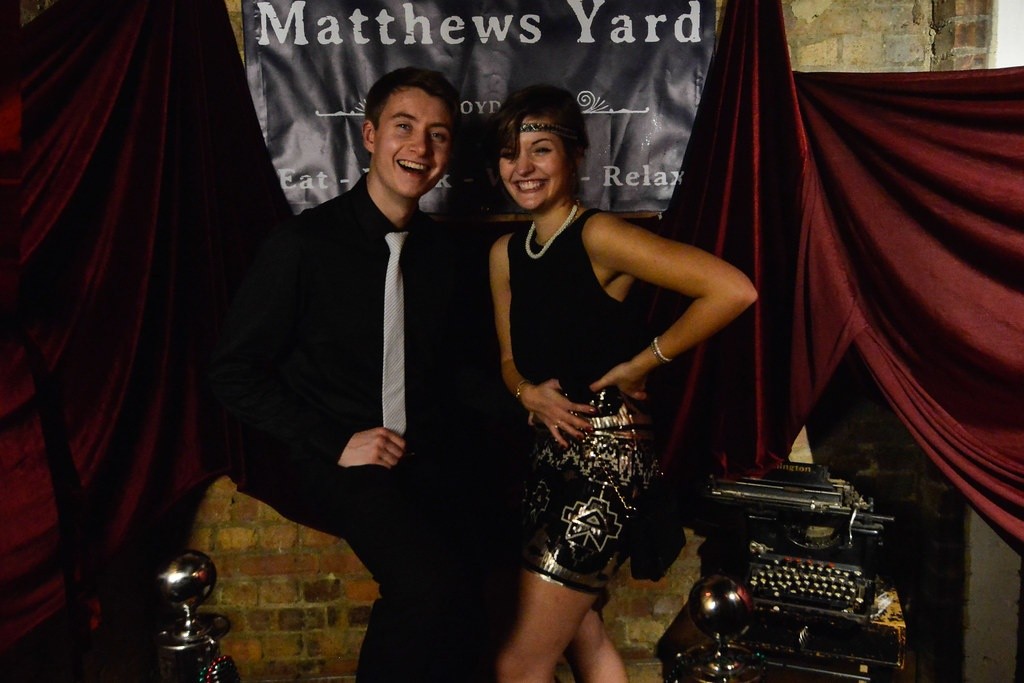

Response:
(237, 66), (522, 682)
(488, 84), (758, 682)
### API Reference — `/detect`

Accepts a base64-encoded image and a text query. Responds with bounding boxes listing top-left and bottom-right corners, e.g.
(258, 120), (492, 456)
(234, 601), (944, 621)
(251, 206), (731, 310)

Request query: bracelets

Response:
(516, 380), (531, 399)
(649, 335), (672, 365)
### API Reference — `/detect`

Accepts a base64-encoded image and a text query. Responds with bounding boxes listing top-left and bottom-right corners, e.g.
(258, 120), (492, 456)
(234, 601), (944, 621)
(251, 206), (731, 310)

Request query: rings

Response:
(556, 419), (563, 427)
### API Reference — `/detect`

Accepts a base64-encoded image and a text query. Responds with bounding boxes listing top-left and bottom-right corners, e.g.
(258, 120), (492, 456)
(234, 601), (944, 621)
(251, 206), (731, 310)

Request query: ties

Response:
(382, 231), (407, 436)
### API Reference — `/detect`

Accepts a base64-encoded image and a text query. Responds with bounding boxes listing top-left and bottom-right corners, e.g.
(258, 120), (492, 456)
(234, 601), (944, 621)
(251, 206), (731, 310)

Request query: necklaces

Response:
(525, 199), (580, 259)
